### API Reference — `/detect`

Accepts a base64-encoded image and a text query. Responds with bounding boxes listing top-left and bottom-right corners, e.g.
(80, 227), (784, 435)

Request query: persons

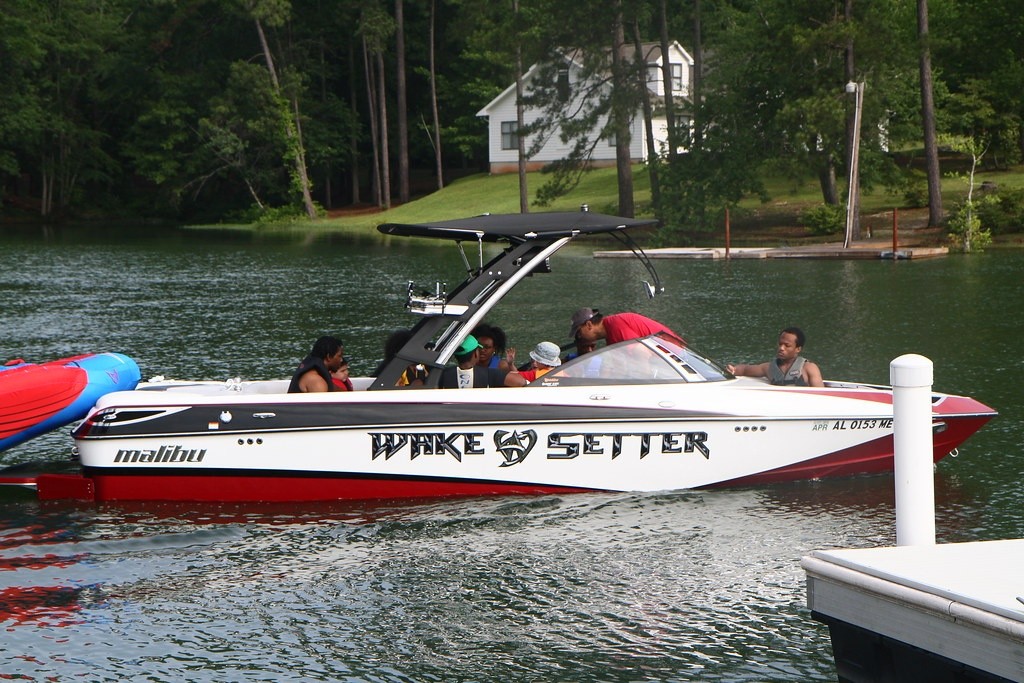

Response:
(288, 335), (354, 392)
(438, 307), (684, 388)
(373, 328), (429, 386)
(725, 327), (824, 387)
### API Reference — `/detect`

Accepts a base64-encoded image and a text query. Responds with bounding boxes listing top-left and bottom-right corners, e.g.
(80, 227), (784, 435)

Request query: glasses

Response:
(576, 344), (596, 349)
(531, 359), (535, 363)
(484, 345), (493, 349)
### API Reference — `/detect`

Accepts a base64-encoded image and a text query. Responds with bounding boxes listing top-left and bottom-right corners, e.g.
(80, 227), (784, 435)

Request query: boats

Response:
(0, 208), (1001, 511)
(0, 351), (141, 451)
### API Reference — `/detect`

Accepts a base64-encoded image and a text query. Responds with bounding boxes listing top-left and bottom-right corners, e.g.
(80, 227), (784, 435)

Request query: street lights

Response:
(844, 81), (866, 248)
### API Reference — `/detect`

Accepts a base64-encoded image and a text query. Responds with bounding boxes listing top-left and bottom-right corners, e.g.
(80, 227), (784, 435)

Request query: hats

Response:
(568, 307), (597, 338)
(453, 335), (483, 356)
(529, 342), (561, 367)
